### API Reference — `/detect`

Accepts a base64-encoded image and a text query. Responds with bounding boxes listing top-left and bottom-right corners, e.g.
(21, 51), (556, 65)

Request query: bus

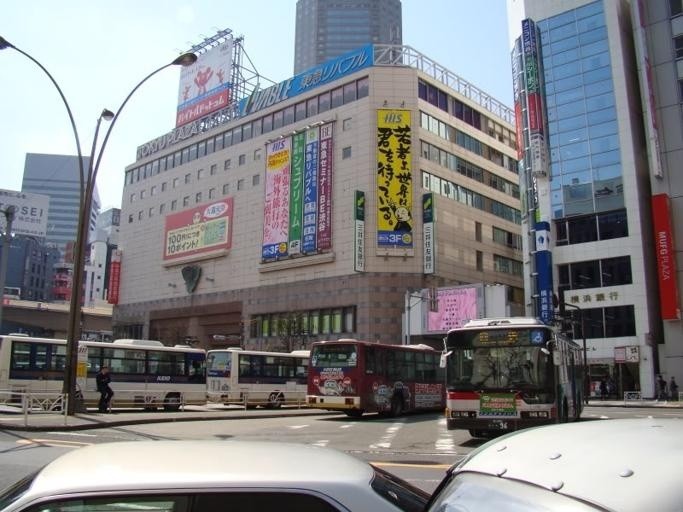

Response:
(2, 286), (20, 300)
(0, 332), (207, 410)
(440, 316), (591, 438)
(590, 375), (609, 397)
(306, 338), (446, 417)
(206, 348), (309, 408)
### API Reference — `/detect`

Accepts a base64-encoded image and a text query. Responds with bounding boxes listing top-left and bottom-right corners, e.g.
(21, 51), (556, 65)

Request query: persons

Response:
(97, 366), (114, 411)
(658, 375), (668, 401)
(670, 376), (679, 400)
(599, 379), (608, 400)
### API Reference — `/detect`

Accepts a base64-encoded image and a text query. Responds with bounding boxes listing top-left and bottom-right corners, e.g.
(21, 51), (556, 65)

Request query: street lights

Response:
(1, 36), (197, 414)
(213, 334), (243, 348)
